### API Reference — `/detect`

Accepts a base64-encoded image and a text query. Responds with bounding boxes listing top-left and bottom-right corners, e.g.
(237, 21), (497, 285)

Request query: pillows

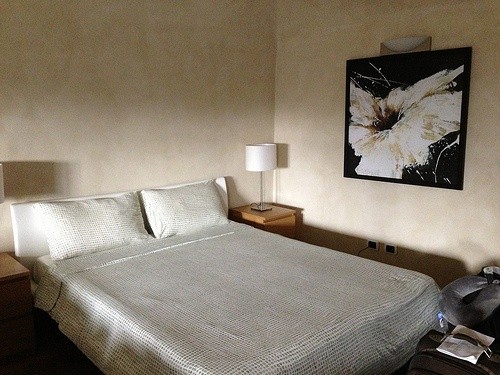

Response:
(34, 192), (155, 260)
(140, 179), (229, 238)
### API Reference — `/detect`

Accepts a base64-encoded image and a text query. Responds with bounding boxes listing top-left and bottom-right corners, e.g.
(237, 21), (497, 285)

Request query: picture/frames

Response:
(344, 47), (472, 190)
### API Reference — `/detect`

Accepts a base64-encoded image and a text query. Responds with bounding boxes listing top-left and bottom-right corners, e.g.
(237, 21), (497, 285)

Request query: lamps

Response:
(246, 144), (277, 212)
(0, 164), (5, 203)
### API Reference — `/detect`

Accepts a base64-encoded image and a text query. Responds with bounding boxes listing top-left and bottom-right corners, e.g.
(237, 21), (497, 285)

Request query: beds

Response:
(11, 176), (448, 375)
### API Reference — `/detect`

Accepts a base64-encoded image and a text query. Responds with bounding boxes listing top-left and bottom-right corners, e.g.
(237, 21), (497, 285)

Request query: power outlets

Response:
(385, 244), (397, 254)
(367, 240), (380, 251)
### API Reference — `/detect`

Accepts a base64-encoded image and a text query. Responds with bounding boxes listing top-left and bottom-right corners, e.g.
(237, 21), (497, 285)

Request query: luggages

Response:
(410, 329), (500, 375)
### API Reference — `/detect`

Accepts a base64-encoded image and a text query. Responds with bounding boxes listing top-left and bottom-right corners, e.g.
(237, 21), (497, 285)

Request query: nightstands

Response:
(0, 250), (35, 361)
(228, 203), (297, 239)
